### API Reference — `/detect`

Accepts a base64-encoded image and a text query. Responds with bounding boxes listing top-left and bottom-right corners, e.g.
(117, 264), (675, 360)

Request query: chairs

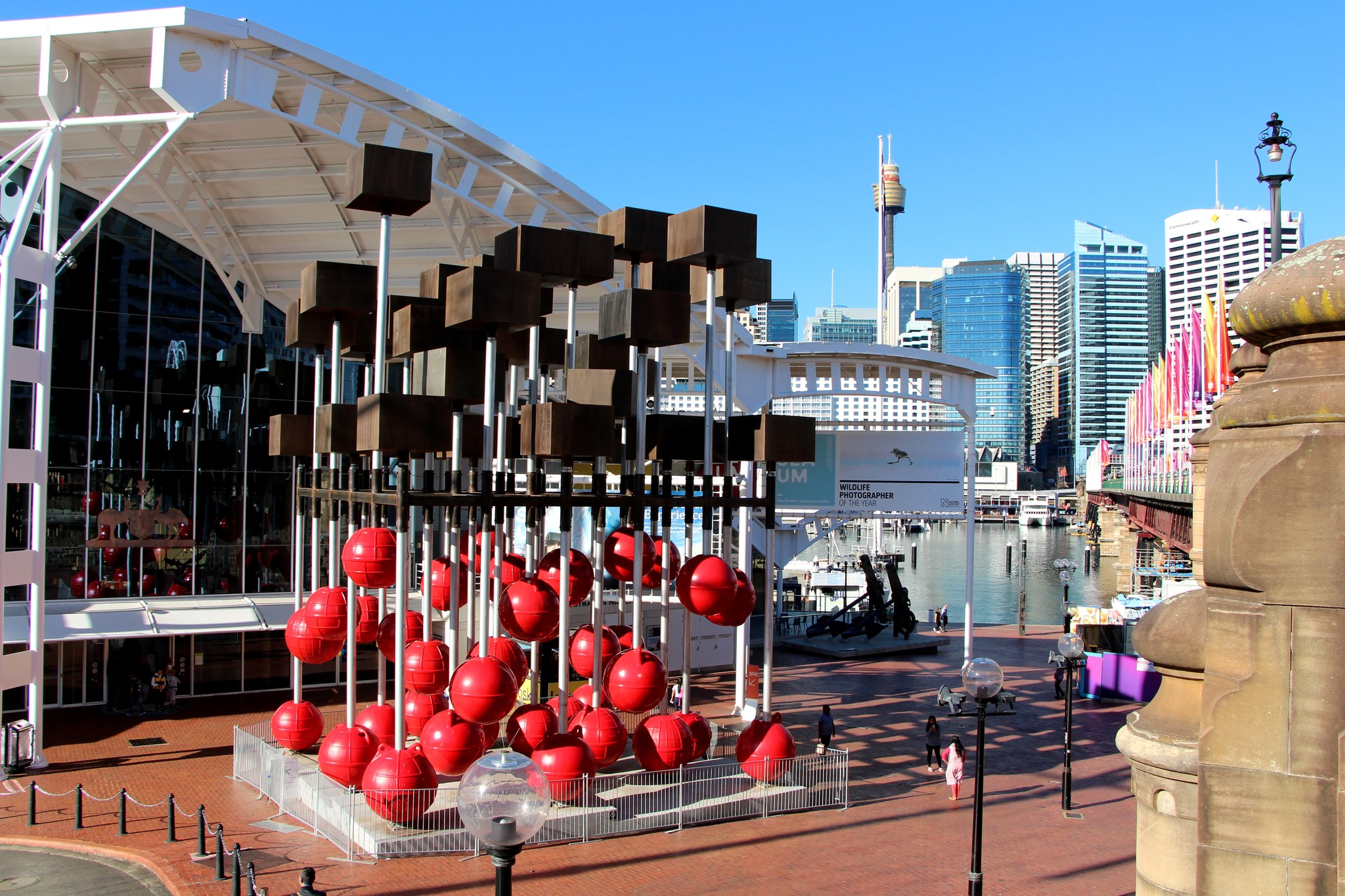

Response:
(773, 613), (808, 634)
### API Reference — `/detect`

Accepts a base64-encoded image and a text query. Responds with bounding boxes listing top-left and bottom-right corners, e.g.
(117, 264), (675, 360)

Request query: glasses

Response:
(825, 708), (830, 710)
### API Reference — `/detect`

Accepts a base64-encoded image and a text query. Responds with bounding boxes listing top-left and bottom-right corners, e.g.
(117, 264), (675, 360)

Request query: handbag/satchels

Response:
(940, 615), (943, 620)
(815, 738), (825, 754)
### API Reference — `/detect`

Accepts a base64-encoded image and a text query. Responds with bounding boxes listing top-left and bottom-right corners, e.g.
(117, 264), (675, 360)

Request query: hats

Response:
(170, 670), (175, 673)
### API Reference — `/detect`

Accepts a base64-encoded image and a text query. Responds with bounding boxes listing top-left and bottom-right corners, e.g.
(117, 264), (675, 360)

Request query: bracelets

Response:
(935, 620), (936, 620)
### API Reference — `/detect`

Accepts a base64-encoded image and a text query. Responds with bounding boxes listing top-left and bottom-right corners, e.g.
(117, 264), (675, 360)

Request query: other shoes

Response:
(155, 710), (159, 713)
(939, 626), (943, 631)
(139, 711), (146, 715)
(159, 709), (165, 713)
(126, 713), (132, 716)
(948, 796), (958, 800)
(1054, 695), (1060, 699)
(169, 703), (179, 710)
(1061, 693), (1065, 697)
(937, 631), (941, 633)
(933, 628), (936, 632)
(943, 630), (948, 632)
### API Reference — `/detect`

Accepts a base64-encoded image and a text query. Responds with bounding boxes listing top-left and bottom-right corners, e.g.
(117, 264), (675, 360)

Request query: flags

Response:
(1124, 265), (1236, 446)
(1100, 438), (1110, 466)
(1058, 466), (1067, 477)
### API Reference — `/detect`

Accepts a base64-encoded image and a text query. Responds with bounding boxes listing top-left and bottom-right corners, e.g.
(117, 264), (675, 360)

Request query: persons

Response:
(1175, 562), (1188, 578)
(670, 678), (682, 712)
(134, 656), (152, 705)
(151, 657), (182, 713)
(126, 673), (146, 716)
(1079, 512), (1085, 516)
(818, 705), (835, 761)
(1054, 662), (1065, 698)
(933, 604), (948, 633)
(941, 735), (966, 800)
(925, 715), (945, 772)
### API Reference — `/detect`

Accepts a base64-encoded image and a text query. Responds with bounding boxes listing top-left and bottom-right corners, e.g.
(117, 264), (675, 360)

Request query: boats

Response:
(852, 511), (890, 605)
(1066, 522), (1087, 534)
(1110, 594), (1149, 609)
(1019, 501), (1051, 526)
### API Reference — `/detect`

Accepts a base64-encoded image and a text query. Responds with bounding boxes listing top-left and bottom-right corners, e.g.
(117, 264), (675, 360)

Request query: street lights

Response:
(962, 656), (1003, 896)
(1254, 113), (1297, 265)
(456, 751), (551, 896)
(1080, 466), (1083, 478)
(1057, 632), (1084, 804)
(1060, 600), (1078, 650)
(1075, 503), (1080, 516)
(1059, 570), (1073, 628)
(834, 555), (858, 611)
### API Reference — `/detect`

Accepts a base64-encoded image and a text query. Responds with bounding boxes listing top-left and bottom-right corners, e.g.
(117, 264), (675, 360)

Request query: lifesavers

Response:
(1077, 530), (1082, 534)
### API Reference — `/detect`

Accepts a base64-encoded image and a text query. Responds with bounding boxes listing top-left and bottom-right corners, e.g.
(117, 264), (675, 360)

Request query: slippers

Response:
(928, 768), (933, 772)
(939, 767), (945, 772)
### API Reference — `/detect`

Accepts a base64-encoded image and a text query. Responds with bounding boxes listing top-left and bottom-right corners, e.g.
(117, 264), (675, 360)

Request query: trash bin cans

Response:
(1, 719), (34, 774)
(929, 609), (934, 622)
